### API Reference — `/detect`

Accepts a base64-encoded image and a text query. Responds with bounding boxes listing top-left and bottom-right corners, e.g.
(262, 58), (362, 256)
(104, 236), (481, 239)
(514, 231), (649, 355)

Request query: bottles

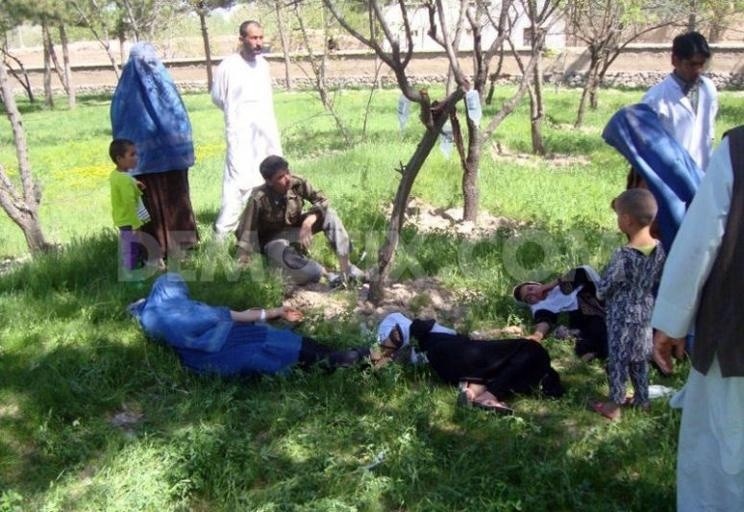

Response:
(439, 123), (455, 163)
(649, 384), (674, 400)
(397, 92), (412, 133)
(464, 88), (483, 130)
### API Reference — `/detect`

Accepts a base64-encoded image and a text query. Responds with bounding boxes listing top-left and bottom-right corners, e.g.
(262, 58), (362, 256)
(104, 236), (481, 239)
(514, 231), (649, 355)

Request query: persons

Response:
(376, 312), (566, 414)
(641, 32), (719, 171)
(512, 265), (609, 366)
(127, 272), (381, 384)
(589, 189), (666, 421)
(602, 102), (704, 254)
(211, 20), (283, 242)
(235, 154), (367, 286)
(110, 42), (201, 254)
(109, 139), (152, 273)
(650, 122), (744, 511)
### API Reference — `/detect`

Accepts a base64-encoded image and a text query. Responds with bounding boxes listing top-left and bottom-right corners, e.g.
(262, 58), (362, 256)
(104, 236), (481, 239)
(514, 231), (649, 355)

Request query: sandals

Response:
(472, 397), (515, 416)
(456, 387), (475, 408)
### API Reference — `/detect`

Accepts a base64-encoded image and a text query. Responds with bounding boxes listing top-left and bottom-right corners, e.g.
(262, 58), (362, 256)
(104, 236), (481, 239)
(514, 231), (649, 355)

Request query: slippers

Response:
(592, 401), (614, 420)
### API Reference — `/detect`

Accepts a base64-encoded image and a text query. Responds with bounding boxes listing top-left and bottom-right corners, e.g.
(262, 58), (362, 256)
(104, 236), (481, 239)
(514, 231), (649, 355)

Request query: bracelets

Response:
(260, 307), (267, 322)
